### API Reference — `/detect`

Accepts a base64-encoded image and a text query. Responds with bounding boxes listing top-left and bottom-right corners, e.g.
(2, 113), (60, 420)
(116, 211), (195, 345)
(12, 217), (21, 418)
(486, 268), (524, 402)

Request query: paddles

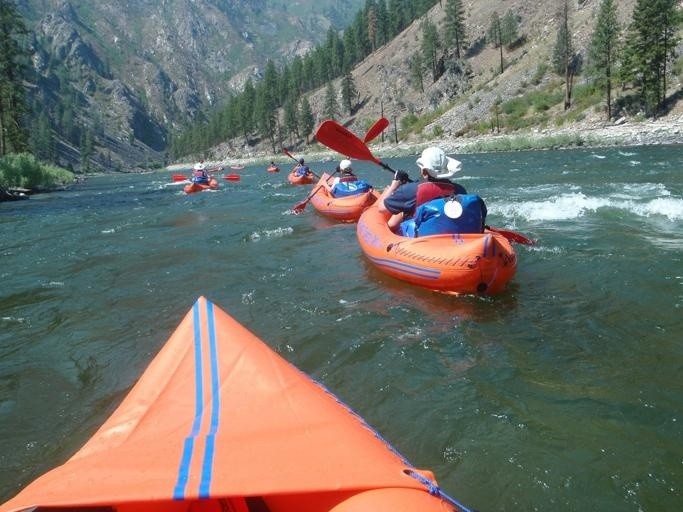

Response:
(317, 121), (532, 245)
(293, 118), (390, 212)
(283, 149), (320, 178)
(173, 174), (240, 181)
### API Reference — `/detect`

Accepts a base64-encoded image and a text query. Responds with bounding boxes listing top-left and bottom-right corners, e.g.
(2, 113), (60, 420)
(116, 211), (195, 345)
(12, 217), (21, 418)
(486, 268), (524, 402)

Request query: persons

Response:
(319, 159), (356, 197)
(192, 167), (208, 185)
(270, 162), (276, 169)
(373, 146), (470, 234)
(290, 158), (309, 177)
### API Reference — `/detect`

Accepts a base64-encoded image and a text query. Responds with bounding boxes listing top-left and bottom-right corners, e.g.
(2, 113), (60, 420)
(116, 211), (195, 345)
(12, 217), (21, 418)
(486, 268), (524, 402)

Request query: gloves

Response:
(394, 168), (408, 184)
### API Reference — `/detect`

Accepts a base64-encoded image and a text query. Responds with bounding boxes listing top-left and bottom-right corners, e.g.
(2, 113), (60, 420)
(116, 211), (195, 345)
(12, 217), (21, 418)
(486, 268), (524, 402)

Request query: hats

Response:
(340, 160), (351, 171)
(299, 158), (304, 163)
(416, 147), (461, 179)
(194, 163), (205, 169)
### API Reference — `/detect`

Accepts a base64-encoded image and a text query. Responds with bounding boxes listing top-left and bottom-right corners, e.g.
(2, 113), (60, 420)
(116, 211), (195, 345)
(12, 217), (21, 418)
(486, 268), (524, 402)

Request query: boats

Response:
(266, 166), (279, 172)
(231, 166), (244, 171)
(182, 177), (217, 193)
(357, 183), (519, 297)
(286, 165), (315, 184)
(311, 171), (379, 221)
(2, 291), (475, 511)
